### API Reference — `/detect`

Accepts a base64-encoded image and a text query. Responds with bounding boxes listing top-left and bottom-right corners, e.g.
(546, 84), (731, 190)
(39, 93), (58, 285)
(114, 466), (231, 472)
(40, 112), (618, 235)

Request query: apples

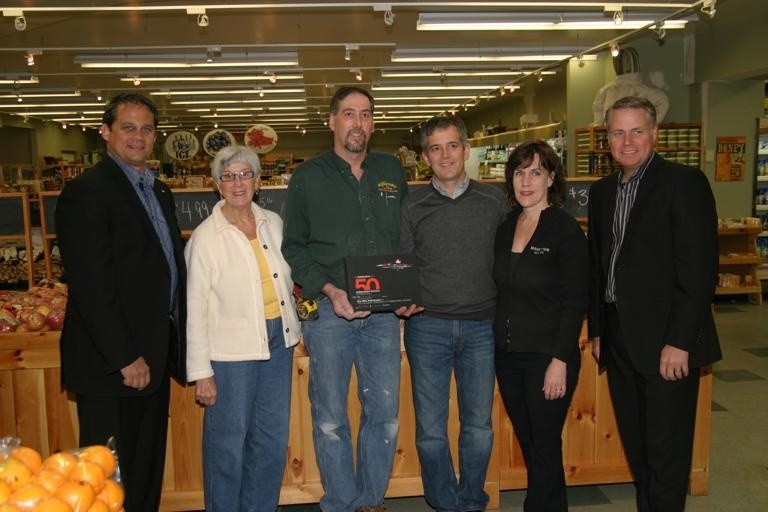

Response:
(0, 281), (68, 334)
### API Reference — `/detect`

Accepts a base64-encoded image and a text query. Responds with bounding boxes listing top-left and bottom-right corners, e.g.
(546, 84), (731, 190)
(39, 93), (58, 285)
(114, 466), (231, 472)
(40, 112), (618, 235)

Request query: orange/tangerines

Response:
(0, 445), (127, 512)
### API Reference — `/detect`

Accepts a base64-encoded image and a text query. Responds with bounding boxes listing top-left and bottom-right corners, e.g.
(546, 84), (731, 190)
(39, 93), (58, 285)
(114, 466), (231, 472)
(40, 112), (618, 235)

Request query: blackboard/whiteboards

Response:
(42, 176), (600, 238)
(0, 192), (30, 240)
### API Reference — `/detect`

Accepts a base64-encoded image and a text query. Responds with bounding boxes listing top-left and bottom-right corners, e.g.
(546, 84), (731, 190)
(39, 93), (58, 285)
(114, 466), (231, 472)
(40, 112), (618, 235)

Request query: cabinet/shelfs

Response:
(573, 126), (703, 182)
(751, 116), (767, 300)
(41, 148), (311, 191)
(0, 287), (711, 509)
(712, 216), (765, 308)
(407, 122), (567, 183)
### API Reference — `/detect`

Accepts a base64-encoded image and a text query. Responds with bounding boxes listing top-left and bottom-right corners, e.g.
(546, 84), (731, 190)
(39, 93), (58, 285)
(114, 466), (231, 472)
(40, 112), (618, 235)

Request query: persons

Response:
(184, 143), (300, 511)
(584, 73), (721, 511)
(53, 92), (185, 512)
(396, 114), (511, 512)
(495, 141), (587, 512)
(280, 85), (411, 511)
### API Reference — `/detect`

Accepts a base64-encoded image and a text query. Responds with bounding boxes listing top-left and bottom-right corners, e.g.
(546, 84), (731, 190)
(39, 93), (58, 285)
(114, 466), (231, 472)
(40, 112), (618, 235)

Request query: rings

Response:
(555, 390), (562, 394)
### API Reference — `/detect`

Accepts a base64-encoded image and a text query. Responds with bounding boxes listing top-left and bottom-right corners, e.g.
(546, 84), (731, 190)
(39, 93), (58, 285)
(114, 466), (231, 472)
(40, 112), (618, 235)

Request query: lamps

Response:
(73, 52), (312, 126)
(0, 67), (180, 131)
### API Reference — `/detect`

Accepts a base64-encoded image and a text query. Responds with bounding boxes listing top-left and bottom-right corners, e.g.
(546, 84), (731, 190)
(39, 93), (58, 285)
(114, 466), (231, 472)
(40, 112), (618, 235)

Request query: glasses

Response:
(219, 170), (254, 182)
